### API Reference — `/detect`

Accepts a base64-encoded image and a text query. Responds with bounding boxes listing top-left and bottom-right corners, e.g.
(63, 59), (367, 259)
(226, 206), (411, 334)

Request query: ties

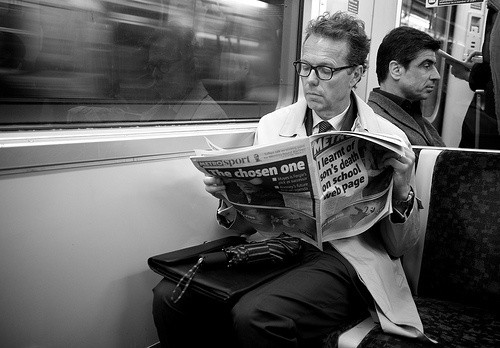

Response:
(319, 121), (332, 132)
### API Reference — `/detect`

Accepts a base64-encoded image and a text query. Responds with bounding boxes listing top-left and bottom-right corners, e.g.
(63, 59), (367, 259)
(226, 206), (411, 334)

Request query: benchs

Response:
(321, 145), (500, 348)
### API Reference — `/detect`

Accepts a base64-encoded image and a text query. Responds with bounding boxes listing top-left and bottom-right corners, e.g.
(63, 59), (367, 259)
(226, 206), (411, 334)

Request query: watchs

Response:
(392, 186), (414, 208)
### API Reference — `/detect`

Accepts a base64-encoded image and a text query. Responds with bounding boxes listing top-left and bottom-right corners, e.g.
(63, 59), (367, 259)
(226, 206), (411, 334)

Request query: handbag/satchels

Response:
(148, 236), (324, 302)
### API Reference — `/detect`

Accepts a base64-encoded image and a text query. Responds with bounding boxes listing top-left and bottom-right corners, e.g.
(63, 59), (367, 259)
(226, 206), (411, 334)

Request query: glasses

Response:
(293, 59), (357, 81)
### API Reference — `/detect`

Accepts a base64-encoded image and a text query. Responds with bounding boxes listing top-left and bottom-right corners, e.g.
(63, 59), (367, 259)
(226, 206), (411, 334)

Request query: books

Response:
(436, 49), (473, 69)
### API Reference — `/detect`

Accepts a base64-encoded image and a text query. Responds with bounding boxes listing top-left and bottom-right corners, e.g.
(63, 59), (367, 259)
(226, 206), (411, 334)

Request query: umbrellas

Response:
(171, 235), (302, 304)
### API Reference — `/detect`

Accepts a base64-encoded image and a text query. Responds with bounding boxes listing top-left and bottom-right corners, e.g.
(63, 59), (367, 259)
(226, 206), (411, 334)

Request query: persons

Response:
(366, 26), (447, 176)
(446, 0), (500, 150)
(152, 10), (424, 348)
